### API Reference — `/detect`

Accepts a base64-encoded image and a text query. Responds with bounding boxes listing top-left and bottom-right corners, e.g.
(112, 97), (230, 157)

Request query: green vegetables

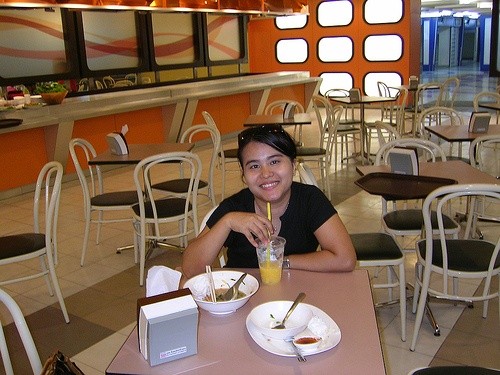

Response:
(33, 81), (66, 93)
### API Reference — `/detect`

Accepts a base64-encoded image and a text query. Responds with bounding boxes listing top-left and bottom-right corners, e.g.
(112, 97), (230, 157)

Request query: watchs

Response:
(282, 256), (290, 269)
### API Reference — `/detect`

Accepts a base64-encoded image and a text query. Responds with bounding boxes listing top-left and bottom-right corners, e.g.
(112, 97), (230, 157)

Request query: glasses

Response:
(238, 123), (283, 144)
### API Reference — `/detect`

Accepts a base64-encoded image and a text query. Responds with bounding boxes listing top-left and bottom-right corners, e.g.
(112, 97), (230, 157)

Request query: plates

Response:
(19, 103), (48, 108)
(245, 303), (340, 358)
(294, 337), (322, 348)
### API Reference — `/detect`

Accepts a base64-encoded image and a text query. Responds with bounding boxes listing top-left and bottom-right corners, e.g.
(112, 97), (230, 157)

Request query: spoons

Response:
(272, 292), (306, 329)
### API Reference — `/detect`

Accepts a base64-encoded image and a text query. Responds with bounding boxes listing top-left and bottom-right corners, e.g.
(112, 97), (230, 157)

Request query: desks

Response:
(480, 104), (500, 111)
(425, 124), (500, 240)
(106, 266), (385, 375)
(355, 160), (500, 336)
(88, 143), (194, 268)
(331, 97), (396, 165)
(401, 85), (440, 138)
(244, 113), (311, 127)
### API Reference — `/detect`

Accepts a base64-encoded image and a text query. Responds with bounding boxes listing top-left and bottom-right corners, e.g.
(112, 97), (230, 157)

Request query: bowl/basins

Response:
(183, 270), (260, 314)
(251, 301), (313, 338)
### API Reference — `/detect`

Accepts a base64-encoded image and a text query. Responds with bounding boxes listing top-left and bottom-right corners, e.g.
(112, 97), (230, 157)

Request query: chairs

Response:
(0, 74), (500, 375)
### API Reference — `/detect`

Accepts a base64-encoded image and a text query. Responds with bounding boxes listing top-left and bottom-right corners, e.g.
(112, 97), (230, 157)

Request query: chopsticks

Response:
(206, 264), (217, 302)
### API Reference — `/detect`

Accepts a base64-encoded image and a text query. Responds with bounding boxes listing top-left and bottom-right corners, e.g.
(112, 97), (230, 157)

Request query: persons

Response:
(181, 124), (356, 280)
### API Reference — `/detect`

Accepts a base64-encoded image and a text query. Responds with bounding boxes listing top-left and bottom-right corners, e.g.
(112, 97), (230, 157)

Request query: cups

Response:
(254, 235), (286, 284)
(25, 94), (31, 103)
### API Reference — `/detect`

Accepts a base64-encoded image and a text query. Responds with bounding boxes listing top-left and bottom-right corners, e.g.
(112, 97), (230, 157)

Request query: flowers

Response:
(36, 81), (67, 93)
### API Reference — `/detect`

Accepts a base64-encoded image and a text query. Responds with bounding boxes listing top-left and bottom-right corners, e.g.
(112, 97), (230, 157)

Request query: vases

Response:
(40, 91), (67, 104)
